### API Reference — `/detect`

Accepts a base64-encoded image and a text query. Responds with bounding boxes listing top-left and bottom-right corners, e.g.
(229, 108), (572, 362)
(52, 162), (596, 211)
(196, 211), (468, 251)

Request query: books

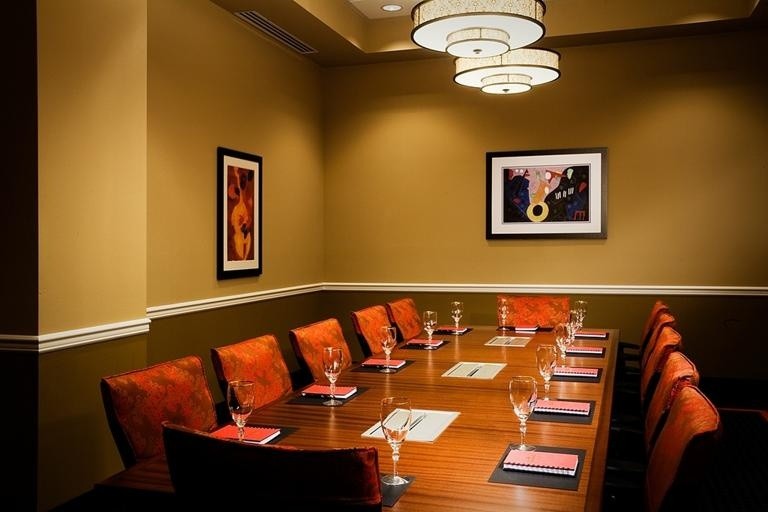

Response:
(554, 366), (599, 377)
(361, 358), (407, 369)
(301, 384), (358, 399)
(502, 448), (580, 476)
(534, 399), (592, 416)
(575, 331), (607, 338)
(566, 346), (603, 355)
(515, 327), (538, 330)
(437, 325), (468, 333)
(210, 426), (282, 445)
(406, 338), (444, 347)
(516, 331), (537, 334)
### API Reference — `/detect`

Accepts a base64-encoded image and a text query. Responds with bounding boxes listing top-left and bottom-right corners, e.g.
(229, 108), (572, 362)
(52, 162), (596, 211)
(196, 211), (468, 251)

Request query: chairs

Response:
(352, 304), (398, 357)
(101, 354), (219, 468)
(386, 297), (423, 342)
(497, 294), (571, 328)
(289, 317), (353, 384)
(210, 334), (293, 417)
(601, 300), (721, 512)
(161, 420), (383, 512)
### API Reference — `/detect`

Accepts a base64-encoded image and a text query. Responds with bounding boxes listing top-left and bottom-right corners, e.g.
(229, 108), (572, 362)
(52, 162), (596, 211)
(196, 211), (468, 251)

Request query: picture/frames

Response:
(485, 146), (608, 239)
(217, 146), (263, 280)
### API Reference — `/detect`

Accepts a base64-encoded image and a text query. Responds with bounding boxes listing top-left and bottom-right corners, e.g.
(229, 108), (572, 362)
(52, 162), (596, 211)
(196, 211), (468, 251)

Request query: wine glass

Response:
(421, 311), (439, 352)
(497, 298), (513, 332)
(378, 397), (411, 485)
(321, 348), (347, 409)
(450, 302), (465, 336)
(225, 380), (256, 444)
(508, 377), (537, 455)
(536, 301), (589, 398)
(377, 325), (398, 373)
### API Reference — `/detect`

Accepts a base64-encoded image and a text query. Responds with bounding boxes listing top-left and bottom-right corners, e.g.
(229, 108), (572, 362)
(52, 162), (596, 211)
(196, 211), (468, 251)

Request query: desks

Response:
(105, 321), (620, 512)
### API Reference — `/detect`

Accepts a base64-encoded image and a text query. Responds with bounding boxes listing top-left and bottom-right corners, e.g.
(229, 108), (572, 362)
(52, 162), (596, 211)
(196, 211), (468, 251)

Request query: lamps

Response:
(452, 47), (562, 95)
(409, 1), (546, 58)
